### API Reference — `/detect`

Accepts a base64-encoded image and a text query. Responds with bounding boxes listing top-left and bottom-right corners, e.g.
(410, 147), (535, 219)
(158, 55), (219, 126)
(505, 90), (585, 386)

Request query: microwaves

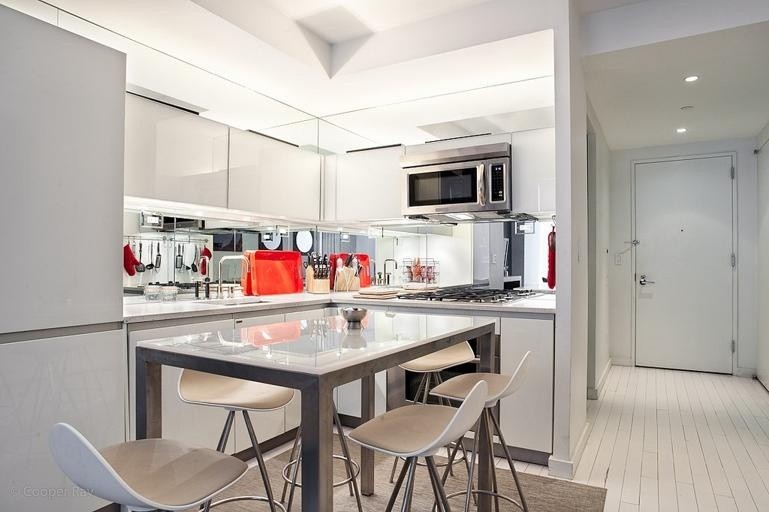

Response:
(403, 157), (511, 220)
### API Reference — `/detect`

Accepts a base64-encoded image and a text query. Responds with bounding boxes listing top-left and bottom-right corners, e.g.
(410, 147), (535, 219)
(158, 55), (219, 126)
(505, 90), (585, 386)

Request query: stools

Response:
(46, 341), (533, 512)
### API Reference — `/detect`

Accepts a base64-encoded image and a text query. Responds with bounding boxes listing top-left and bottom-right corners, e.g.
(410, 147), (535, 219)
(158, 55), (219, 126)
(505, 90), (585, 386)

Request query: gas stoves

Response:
(397, 286), (536, 303)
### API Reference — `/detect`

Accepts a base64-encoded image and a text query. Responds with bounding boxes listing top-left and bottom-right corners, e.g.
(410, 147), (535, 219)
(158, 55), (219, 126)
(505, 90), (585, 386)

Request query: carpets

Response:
(185, 432), (609, 510)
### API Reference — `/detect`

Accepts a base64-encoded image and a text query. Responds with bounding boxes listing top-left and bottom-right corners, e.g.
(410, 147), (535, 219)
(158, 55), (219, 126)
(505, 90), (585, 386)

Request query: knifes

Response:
(309, 253), (329, 279)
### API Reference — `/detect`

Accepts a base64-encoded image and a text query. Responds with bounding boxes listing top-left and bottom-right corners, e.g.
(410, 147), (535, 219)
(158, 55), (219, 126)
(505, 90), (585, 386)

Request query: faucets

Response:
(219, 255), (251, 286)
(199, 256), (210, 276)
(384, 259), (397, 284)
(370, 259), (377, 285)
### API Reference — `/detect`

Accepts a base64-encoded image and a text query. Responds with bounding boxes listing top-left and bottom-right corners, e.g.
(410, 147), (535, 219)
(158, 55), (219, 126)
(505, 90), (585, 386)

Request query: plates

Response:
(296, 230), (314, 253)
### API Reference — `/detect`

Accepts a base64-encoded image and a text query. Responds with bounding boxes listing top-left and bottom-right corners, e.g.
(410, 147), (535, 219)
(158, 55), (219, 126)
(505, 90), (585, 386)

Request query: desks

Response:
(135, 316), (498, 512)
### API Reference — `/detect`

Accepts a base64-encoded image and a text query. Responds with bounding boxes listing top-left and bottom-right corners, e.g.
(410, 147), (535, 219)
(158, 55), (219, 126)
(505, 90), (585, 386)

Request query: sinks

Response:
(193, 297), (274, 306)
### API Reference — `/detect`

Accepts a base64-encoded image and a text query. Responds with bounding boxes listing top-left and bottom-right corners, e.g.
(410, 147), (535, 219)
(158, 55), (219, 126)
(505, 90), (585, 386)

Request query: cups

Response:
(145, 285), (179, 303)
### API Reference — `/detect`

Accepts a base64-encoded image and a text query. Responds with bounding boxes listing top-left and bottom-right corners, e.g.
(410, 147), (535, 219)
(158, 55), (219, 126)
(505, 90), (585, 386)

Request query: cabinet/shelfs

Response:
(124, 90), (323, 225)
(510, 129), (556, 214)
(323, 146), (404, 223)
(127, 304), (555, 465)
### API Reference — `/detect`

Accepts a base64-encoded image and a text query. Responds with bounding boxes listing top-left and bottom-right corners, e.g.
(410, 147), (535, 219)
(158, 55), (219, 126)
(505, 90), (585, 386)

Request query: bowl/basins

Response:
(348, 322), (364, 329)
(341, 307), (367, 322)
